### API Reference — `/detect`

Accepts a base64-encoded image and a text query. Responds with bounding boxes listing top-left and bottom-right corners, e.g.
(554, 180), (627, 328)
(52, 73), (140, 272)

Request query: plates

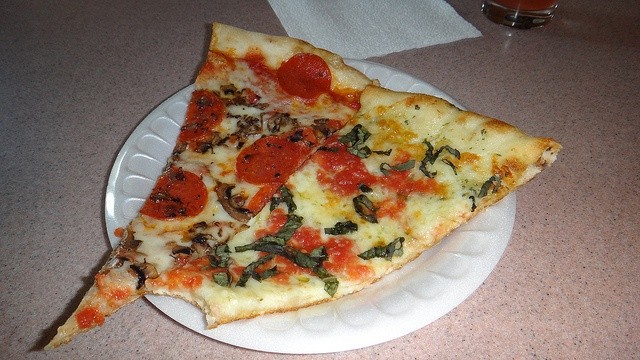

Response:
(103, 56), (518, 356)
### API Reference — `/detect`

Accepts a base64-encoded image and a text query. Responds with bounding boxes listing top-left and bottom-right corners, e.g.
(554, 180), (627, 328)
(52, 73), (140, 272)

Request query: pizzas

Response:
(41, 20), (565, 351)
